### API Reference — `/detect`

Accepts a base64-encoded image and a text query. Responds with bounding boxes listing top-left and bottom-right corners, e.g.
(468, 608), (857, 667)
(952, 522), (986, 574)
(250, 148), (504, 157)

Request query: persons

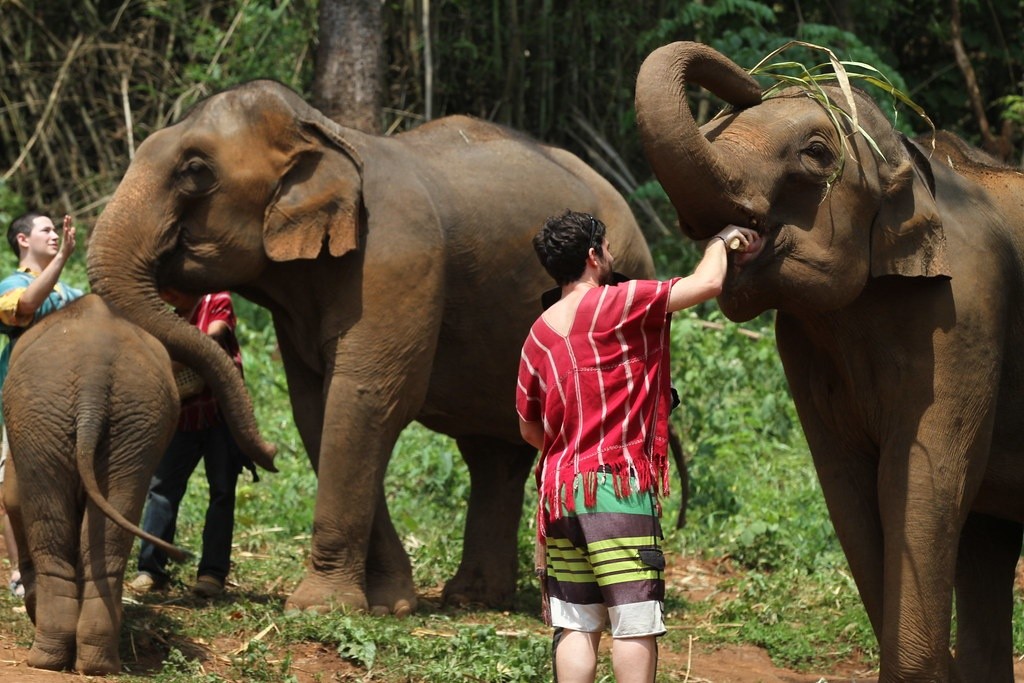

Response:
(0, 211), (86, 598)
(517, 210), (751, 683)
(127, 290), (246, 601)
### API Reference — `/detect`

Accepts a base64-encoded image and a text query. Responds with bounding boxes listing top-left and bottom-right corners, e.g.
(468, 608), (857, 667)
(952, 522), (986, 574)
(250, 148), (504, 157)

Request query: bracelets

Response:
(710, 236), (727, 248)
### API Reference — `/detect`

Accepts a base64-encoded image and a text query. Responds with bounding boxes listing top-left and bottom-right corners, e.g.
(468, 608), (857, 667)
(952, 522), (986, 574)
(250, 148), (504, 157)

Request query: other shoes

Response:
(10, 570), (27, 613)
(193, 580), (223, 599)
(131, 573), (155, 593)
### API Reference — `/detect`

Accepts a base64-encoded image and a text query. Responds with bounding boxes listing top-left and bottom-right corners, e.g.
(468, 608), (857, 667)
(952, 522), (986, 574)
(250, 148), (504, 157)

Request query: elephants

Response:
(0, 292), (195, 672)
(635, 41), (1024, 682)
(87, 76), (690, 617)
